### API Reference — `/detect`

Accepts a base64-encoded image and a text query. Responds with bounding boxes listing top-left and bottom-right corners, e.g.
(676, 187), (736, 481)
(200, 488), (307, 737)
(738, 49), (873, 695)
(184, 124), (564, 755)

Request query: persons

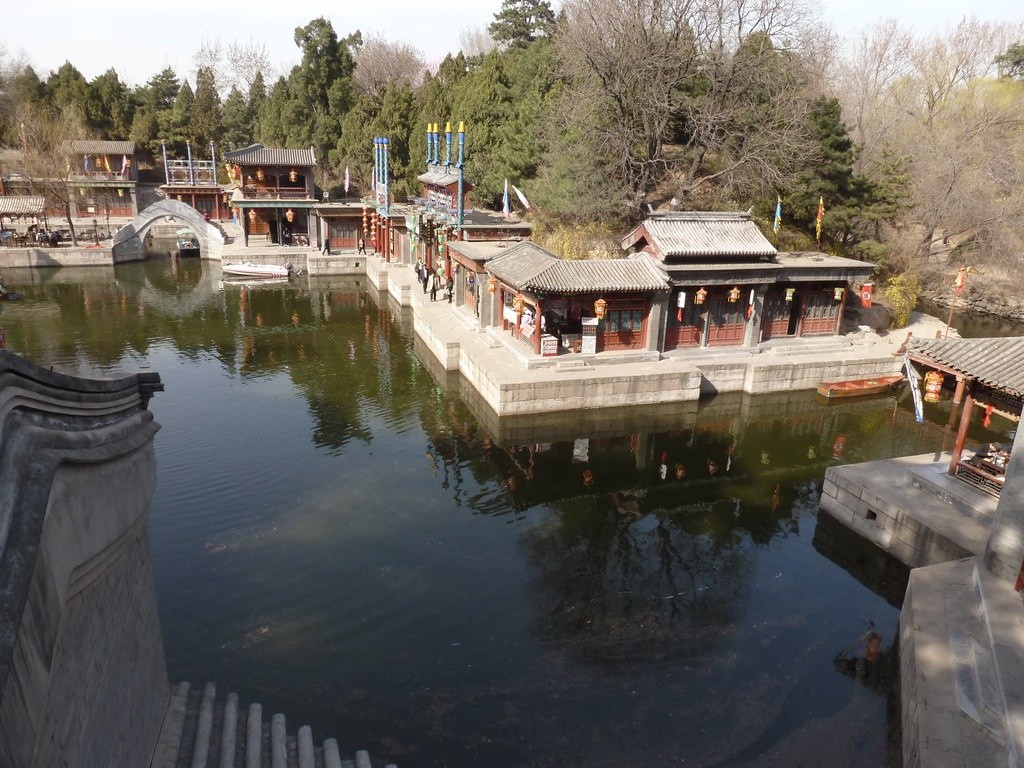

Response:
(414, 257), (423, 284)
(359, 237), (367, 256)
(971, 442), (1001, 468)
(446, 275), (453, 303)
(322, 238), (330, 256)
(427, 270), (440, 302)
(420, 264), (430, 294)
(282, 227), (290, 247)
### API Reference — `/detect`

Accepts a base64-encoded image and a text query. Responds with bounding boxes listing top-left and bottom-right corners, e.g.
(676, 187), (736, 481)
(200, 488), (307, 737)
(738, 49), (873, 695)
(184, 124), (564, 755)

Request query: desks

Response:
(979, 459), (1005, 478)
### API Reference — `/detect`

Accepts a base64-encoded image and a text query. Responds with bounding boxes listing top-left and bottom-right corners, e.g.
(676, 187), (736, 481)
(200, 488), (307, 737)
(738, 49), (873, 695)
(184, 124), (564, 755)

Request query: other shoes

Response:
(448, 301), (451, 304)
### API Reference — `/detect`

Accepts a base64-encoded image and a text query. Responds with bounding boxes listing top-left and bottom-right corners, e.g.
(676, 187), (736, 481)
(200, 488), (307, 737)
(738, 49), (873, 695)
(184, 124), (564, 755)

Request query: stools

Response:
(574, 340), (582, 353)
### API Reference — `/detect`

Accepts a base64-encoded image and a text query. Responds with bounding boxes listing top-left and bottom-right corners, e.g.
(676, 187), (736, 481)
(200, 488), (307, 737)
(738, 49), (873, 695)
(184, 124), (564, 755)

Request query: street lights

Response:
(93, 219), (100, 246)
(105, 214), (112, 238)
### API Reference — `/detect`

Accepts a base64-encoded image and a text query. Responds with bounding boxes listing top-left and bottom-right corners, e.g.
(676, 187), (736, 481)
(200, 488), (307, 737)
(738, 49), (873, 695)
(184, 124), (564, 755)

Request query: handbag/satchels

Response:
(445, 286), (450, 295)
(415, 269), (420, 273)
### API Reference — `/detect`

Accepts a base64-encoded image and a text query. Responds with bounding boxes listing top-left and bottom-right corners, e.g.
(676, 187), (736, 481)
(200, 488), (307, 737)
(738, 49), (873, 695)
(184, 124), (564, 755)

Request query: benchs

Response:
(956, 461), (1004, 497)
(846, 382), (861, 389)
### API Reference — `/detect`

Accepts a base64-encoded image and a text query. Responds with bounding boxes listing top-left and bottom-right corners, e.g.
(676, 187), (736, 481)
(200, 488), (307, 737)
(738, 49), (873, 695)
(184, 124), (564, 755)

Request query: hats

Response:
(993, 442), (1002, 450)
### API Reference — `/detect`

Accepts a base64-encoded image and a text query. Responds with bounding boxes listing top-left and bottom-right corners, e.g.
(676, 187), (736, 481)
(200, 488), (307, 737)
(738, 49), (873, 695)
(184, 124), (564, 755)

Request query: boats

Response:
(176, 228), (199, 256)
(221, 262), (290, 278)
